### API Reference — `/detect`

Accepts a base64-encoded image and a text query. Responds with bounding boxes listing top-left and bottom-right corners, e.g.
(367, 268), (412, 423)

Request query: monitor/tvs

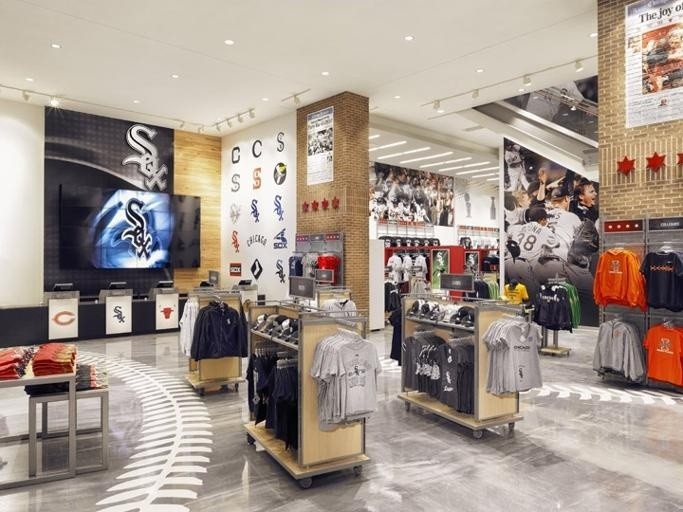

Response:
(107, 281), (127, 289)
(52, 283), (74, 292)
(58, 183), (201, 270)
(238, 279), (253, 285)
(155, 281), (174, 288)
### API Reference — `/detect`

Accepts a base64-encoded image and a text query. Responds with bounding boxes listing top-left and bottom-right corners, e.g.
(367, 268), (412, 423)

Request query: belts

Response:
(543, 257), (559, 261)
(572, 259), (587, 268)
(505, 258), (525, 262)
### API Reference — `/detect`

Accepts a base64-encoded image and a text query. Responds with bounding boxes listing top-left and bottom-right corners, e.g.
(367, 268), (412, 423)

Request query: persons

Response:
(501, 149), (599, 327)
(628, 27), (683, 95)
(365, 162), (457, 226)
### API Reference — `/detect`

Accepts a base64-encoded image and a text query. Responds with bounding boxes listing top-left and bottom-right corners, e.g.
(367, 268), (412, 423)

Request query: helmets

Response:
(374, 166), (453, 208)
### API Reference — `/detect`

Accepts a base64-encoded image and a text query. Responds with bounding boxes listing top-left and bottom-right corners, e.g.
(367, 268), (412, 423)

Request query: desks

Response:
(0, 340), (109, 490)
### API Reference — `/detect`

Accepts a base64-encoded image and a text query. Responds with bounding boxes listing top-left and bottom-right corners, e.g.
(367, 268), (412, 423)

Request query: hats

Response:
(407, 301), (474, 329)
(252, 312), (299, 345)
(527, 207), (552, 221)
(508, 279), (518, 289)
(527, 181), (548, 196)
(551, 188), (571, 198)
